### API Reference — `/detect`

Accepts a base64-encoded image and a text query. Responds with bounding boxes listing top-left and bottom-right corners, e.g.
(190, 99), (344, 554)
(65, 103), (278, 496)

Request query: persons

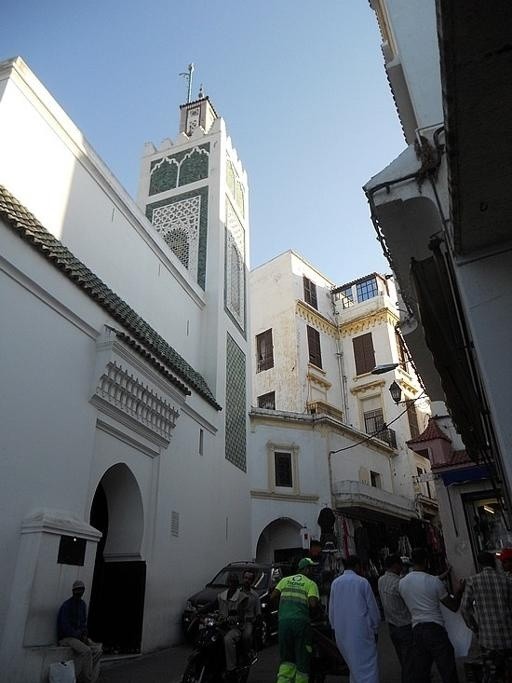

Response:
(57, 580), (102, 682)
(195, 547), (511, 682)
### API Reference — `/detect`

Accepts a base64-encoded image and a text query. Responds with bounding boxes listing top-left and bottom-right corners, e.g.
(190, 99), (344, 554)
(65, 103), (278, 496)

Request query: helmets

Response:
(496, 549), (512, 561)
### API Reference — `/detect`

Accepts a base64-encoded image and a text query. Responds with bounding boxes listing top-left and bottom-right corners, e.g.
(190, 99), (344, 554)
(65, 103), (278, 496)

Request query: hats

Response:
(296, 557), (320, 573)
(72, 580), (85, 591)
(408, 549), (428, 562)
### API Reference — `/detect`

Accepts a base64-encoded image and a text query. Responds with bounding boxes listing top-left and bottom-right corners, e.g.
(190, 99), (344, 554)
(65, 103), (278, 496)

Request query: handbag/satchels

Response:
(49, 660), (76, 683)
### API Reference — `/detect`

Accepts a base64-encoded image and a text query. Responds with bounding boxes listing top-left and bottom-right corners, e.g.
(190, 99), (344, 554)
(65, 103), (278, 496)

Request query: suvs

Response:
(184, 558), (295, 643)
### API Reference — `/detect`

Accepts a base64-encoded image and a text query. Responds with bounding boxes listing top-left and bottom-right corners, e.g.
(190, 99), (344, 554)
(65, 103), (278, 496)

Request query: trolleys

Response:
(308, 601), (350, 682)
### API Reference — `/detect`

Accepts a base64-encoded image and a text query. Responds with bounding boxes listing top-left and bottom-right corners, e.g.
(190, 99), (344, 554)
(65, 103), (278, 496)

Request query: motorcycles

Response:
(180, 611), (268, 681)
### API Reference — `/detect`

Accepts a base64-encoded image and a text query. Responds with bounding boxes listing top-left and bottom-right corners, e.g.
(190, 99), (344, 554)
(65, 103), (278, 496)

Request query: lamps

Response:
(368, 354), (416, 377)
(389, 379), (432, 405)
